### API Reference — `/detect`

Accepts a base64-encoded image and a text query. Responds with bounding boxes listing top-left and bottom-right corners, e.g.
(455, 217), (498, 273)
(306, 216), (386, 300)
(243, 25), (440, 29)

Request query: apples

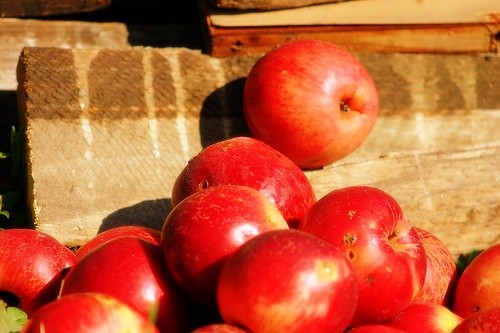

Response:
(243, 40), (378, 168)
(1, 136), (500, 333)
